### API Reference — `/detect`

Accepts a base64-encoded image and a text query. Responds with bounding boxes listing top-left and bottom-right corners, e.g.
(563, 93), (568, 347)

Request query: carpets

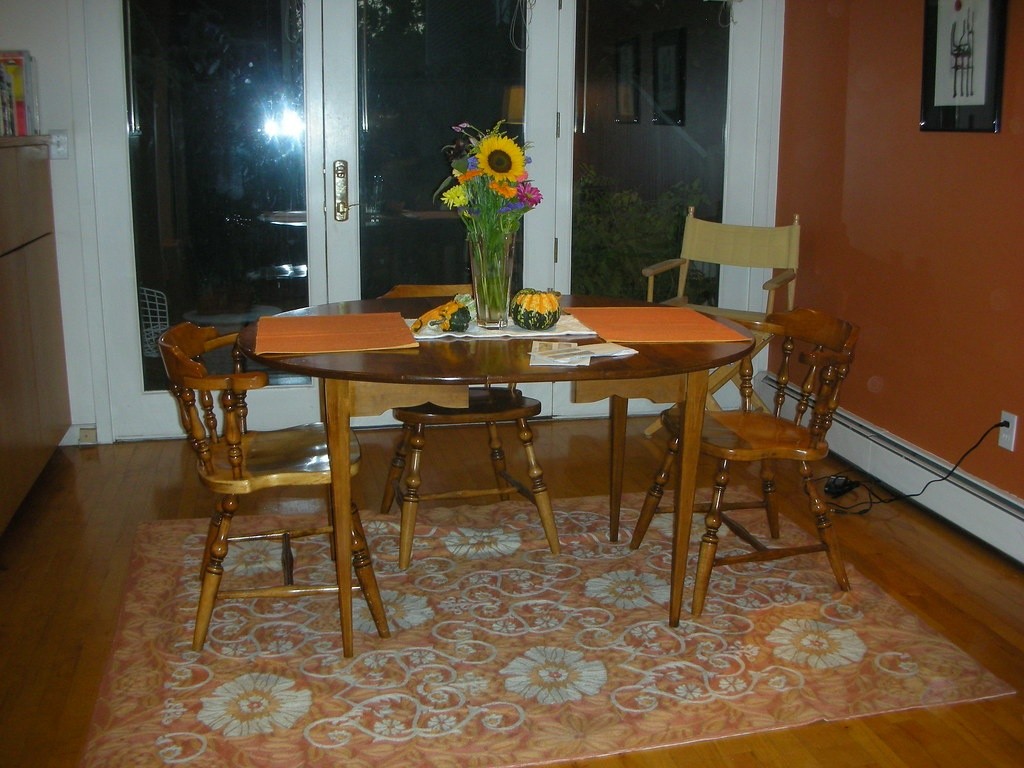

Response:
(79, 485), (1017, 768)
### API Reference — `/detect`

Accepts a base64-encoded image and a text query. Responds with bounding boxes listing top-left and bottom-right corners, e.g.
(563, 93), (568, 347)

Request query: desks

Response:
(257, 206), (462, 233)
(235, 289), (759, 658)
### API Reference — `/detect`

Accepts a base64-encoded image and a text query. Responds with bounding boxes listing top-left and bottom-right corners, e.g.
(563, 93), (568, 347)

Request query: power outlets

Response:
(998, 409), (1019, 452)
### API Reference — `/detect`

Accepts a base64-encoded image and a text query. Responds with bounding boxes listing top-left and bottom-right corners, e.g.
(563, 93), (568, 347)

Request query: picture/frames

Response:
(918, 0), (1009, 133)
(616, 36), (641, 125)
(652, 26), (689, 126)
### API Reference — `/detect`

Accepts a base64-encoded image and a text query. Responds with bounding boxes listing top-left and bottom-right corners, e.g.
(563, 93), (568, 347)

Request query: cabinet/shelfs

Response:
(0, 134), (72, 536)
(129, 55), (194, 312)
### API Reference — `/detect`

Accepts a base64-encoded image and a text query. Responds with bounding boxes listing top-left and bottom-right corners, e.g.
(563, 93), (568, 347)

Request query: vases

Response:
(365, 172), (384, 227)
(466, 233), (516, 329)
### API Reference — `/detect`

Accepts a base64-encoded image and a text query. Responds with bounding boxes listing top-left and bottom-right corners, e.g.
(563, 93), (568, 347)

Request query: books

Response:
(0, 50), (41, 136)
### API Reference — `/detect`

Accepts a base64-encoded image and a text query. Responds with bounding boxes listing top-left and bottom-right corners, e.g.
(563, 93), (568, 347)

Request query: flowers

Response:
(431, 119), (543, 223)
(359, 111), (407, 172)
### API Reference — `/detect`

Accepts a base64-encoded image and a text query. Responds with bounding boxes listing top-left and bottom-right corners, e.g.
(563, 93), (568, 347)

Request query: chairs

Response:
(629, 306), (862, 616)
(159, 321), (390, 658)
(639, 206), (801, 438)
(373, 283), (560, 569)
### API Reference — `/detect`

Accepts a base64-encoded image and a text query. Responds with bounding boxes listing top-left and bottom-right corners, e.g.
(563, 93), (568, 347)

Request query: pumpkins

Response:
(409, 293), (476, 333)
(510, 288), (561, 330)
